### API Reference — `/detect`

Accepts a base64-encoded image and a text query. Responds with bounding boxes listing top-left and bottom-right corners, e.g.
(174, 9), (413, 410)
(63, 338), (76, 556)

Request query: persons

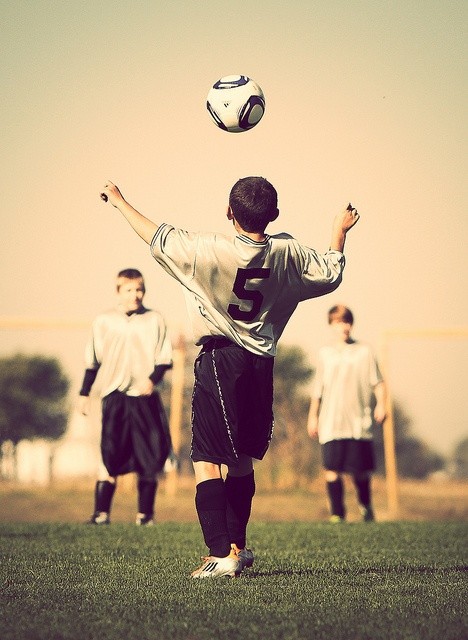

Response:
(77, 269), (173, 528)
(99, 176), (361, 580)
(306, 304), (387, 524)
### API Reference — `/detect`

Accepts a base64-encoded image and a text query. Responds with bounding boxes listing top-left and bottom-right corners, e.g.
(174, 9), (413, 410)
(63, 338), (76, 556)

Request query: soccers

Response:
(206, 75), (267, 131)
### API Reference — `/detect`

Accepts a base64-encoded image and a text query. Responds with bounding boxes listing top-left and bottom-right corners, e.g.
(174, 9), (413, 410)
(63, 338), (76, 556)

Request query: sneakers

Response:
(328, 515), (345, 524)
(231, 544), (253, 566)
(191, 550), (239, 581)
(361, 509), (373, 522)
(84, 511), (110, 524)
(135, 513), (154, 527)
